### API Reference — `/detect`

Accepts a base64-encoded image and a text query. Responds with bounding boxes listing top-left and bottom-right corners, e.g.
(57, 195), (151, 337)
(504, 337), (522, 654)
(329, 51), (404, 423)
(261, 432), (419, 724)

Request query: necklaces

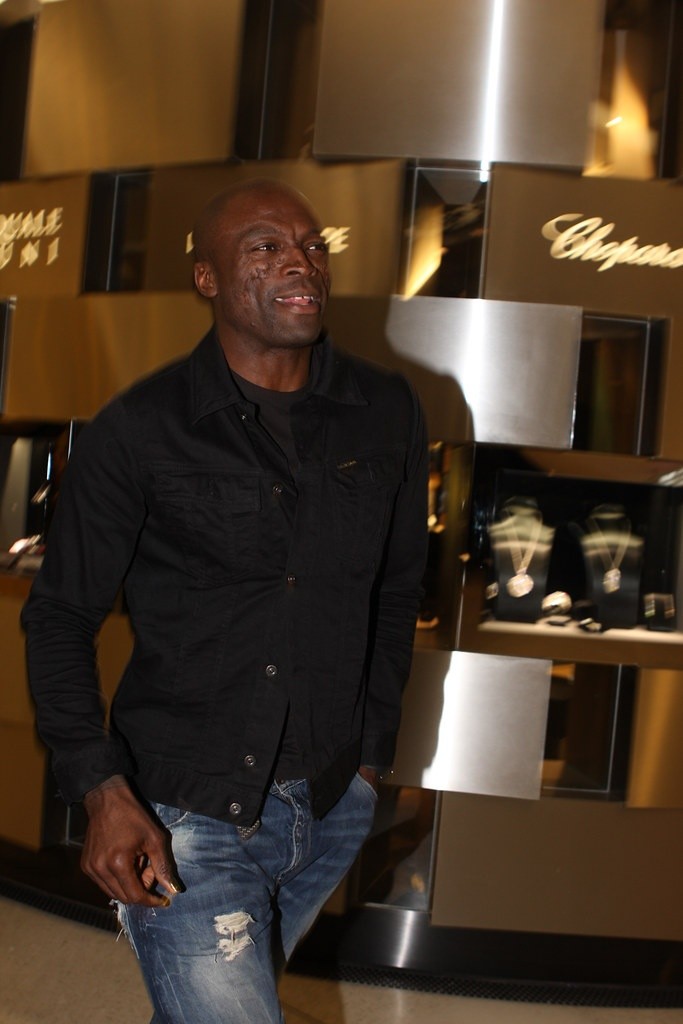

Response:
(504, 517), (544, 597)
(594, 531), (631, 592)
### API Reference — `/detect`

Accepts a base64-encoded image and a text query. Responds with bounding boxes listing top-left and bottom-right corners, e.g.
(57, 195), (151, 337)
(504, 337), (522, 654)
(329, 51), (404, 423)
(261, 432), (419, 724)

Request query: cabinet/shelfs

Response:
(0, 0), (683, 987)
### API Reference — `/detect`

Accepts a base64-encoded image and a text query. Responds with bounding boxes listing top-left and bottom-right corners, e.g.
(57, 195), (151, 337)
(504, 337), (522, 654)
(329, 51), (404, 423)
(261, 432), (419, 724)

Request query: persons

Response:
(18, 179), (430, 1024)
